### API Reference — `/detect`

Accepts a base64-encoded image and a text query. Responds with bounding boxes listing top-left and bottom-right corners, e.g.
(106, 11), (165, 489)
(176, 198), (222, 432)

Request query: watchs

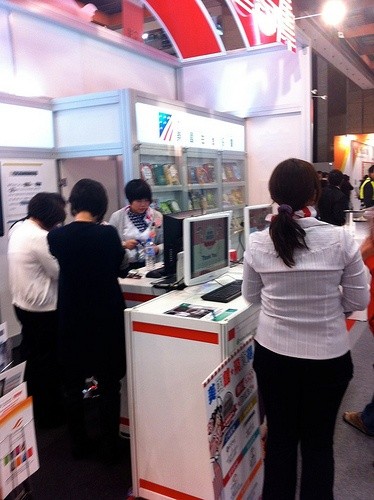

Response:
(121, 241), (127, 249)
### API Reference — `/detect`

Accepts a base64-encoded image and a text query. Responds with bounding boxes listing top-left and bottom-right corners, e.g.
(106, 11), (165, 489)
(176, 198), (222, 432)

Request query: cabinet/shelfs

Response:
(133, 151), (248, 237)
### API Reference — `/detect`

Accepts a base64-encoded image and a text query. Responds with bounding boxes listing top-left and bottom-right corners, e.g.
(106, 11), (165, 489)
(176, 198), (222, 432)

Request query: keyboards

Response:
(201, 279), (244, 304)
(146, 267), (165, 278)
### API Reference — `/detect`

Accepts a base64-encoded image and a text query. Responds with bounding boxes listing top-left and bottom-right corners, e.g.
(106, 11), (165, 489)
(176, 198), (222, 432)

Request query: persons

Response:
(242, 157), (370, 500)
(8, 178), (130, 461)
(316, 169), (354, 227)
(110, 179), (164, 269)
(356, 165), (374, 208)
(342, 210), (374, 434)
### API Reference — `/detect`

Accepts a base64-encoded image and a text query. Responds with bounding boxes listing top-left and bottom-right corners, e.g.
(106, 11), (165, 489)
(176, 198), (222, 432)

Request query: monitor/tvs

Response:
(163, 208), (206, 276)
(243, 204), (274, 253)
(175, 209), (231, 286)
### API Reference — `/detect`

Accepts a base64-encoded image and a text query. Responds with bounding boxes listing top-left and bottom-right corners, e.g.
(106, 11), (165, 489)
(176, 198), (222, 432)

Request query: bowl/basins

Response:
(344, 210), (365, 219)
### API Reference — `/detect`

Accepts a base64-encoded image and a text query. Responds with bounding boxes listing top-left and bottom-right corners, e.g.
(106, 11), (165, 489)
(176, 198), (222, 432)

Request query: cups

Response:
(230, 248), (237, 261)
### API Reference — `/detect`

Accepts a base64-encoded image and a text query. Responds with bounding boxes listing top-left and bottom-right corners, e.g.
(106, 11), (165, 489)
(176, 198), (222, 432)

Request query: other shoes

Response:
(342, 411), (373, 437)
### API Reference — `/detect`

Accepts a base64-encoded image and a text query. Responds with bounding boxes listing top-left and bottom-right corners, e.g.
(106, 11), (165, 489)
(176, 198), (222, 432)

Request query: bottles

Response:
(144, 239), (155, 271)
